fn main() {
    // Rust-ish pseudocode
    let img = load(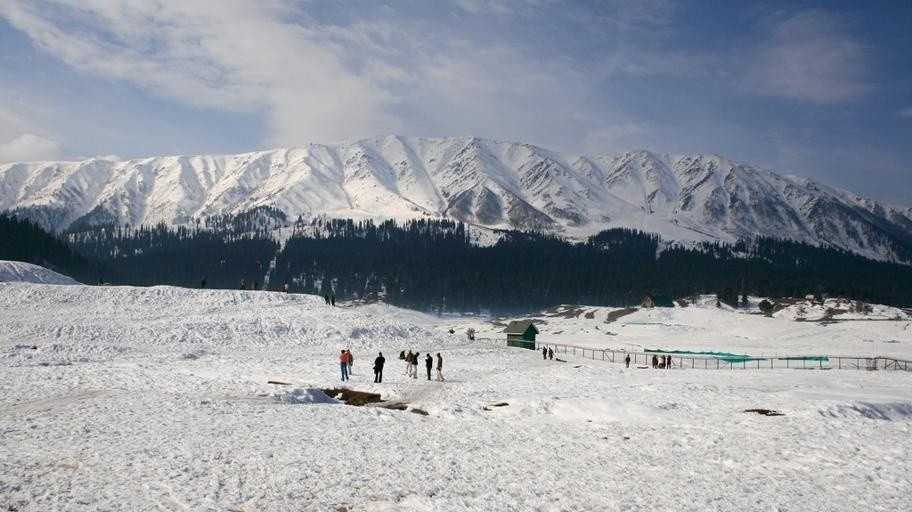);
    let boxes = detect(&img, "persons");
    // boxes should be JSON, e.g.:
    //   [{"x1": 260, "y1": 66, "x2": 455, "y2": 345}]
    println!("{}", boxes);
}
[
  {"x1": 543, "y1": 347, "x2": 547, "y2": 359},
  {"x1": 346, "y1": 349, "x2": 353, "y2": 375},
  {"x1": 374, "y1": 352, "x2": 385, "y2": 383},
  {"x1": 331, "y1": 295, "x2": 335, "y2": 305},
  {"x1": 201, "y1": 278, "x2": 205, "y2": 288},
  {"x1": 238, "y1": 278, "x2": 289, "y2": 293},
  {"x1": 435, "y1": 353, "x2": 444, "y2": 381},
  {"x1": 652, "y1": 355, "x2": 672, "y2": 369},
  {"x1": 625, "y1": 354, "x2": 629, "y2": 368},
  {"x1": 425, "y1": 355, "x2": 432, "y2": 380},
  {"x1": 339, "y1": 350, "x2": 349, "y2": 382},
  {"x1": 324, "y1": 294, "x2": 330, "y2": 305},
  {"x1": 467, "y1": 327, "x2": 475, "y2": 341},
  {"x1": 405, "y1": 350, "x2": 413, "y2": 374},
  {"x1": 409, "y1": 352, "x2": 419, "y2": 379},
  {"x1": 549, "y1": 348, "x2": 553, "y2": 360}
]
